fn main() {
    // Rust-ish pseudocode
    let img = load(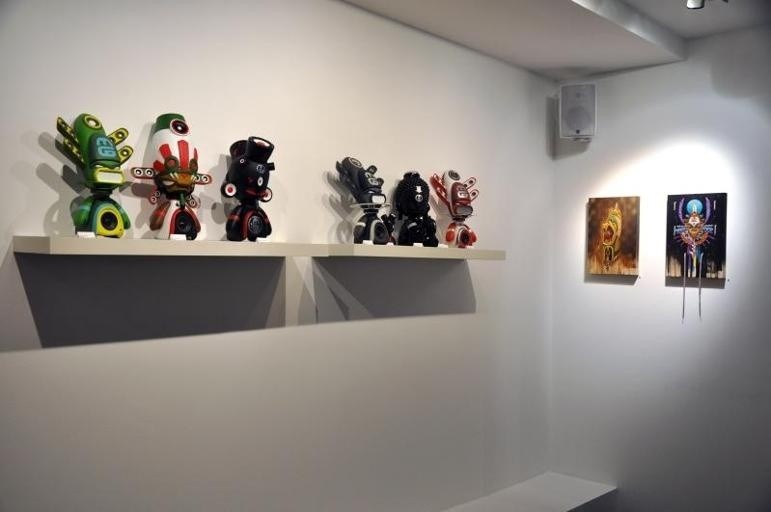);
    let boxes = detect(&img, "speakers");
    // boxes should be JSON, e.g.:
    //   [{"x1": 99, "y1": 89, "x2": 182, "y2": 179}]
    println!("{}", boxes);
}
[{"x1": 559, "y1": 83, "x2": 598, "y2": 140}]
